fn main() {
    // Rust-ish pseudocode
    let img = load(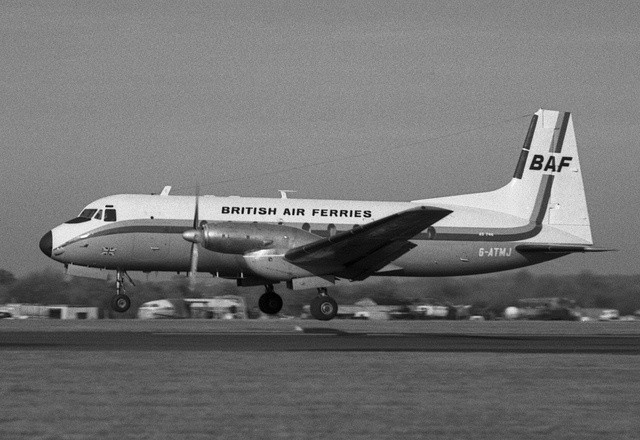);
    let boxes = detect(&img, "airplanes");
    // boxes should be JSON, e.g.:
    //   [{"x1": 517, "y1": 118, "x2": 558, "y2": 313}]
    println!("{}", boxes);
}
[{"x1": 39, "y1": 108, "x2": 617, "y2": 319}]
